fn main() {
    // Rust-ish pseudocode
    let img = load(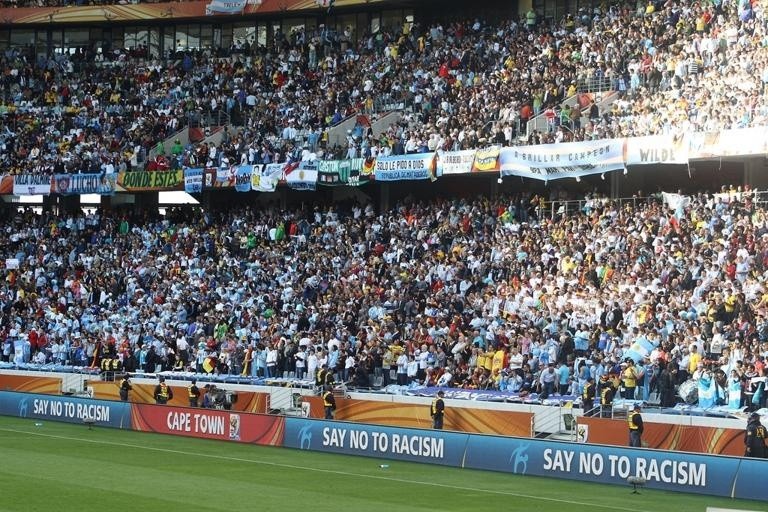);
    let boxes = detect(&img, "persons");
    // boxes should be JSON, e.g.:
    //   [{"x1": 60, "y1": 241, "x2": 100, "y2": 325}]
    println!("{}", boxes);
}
[
  {"x1": 428, "y1": 389, "x2": 446, "y2": 429},
  {"x1": 625, "y1": 404, "x2": 646, "y2": 447},
  {"x1": 1, "y1": 0, "x2": 767, "y2": 413},
  {"x1": 745, "y1": 413, "x2": 768, "y2": 458},
  {"x1": 322, "y1": 386, "x2": 336, "y2": 420}
]
